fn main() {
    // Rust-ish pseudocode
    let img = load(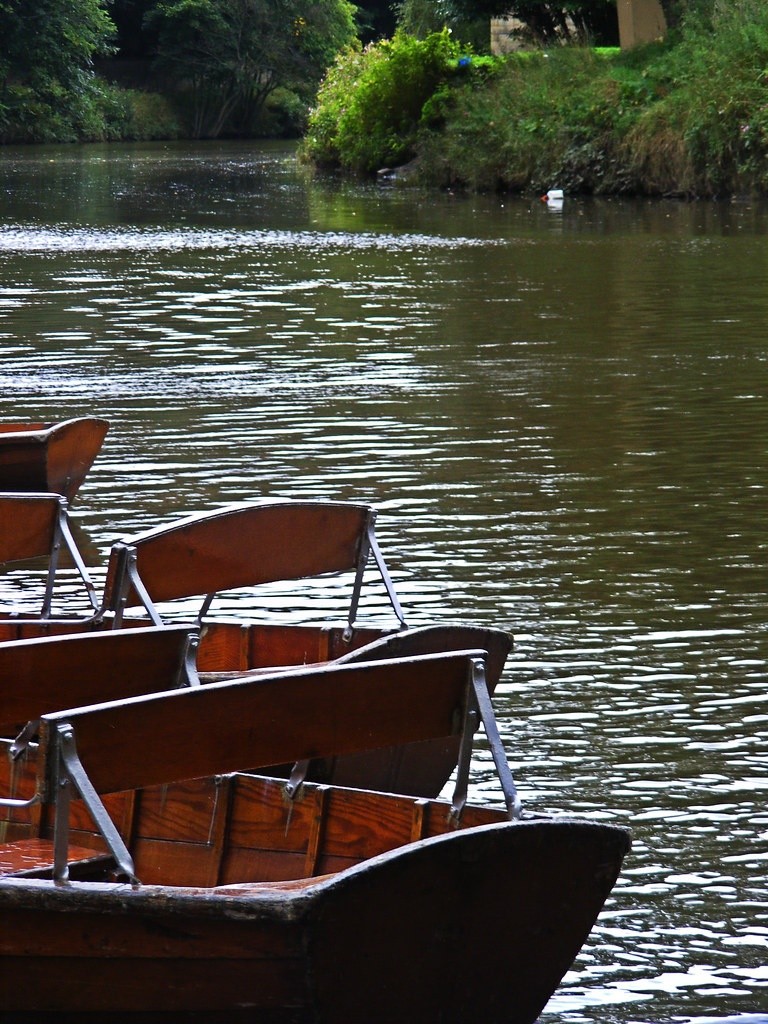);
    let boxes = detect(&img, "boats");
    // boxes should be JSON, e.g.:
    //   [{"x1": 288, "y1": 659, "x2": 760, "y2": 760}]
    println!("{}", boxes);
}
[{"x1": 1, "y1": 416, "x2": 632, "y2": 1024}]
